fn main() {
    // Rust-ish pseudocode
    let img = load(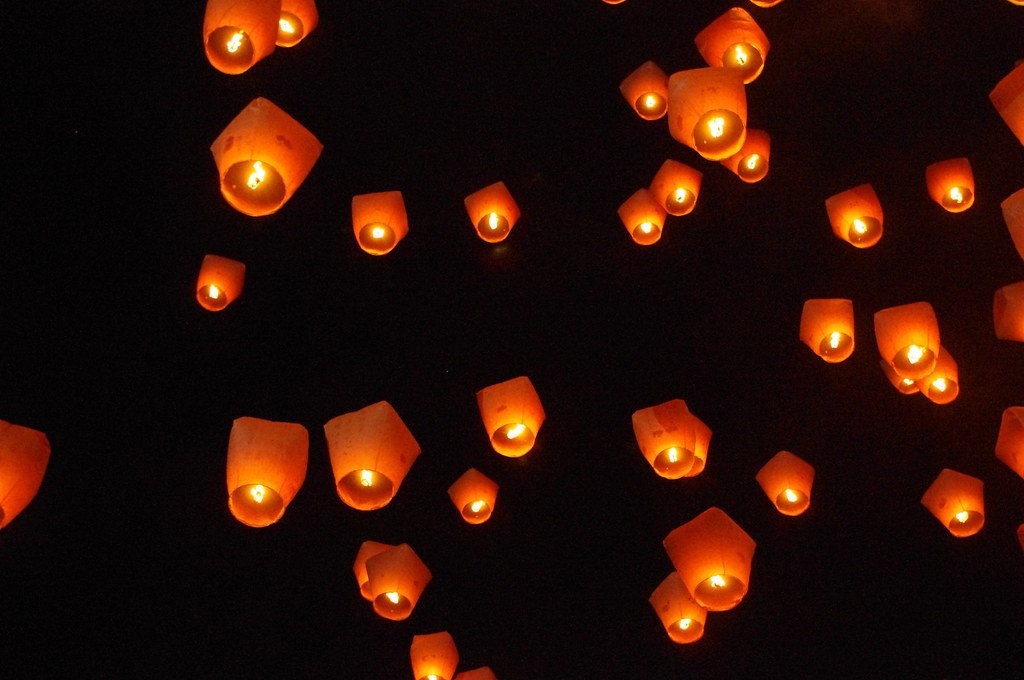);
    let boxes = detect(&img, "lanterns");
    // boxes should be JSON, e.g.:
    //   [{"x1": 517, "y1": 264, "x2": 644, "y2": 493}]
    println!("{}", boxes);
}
[{"x1": 0, "y1": 0, "x2": 1024, "y2": 680}]
